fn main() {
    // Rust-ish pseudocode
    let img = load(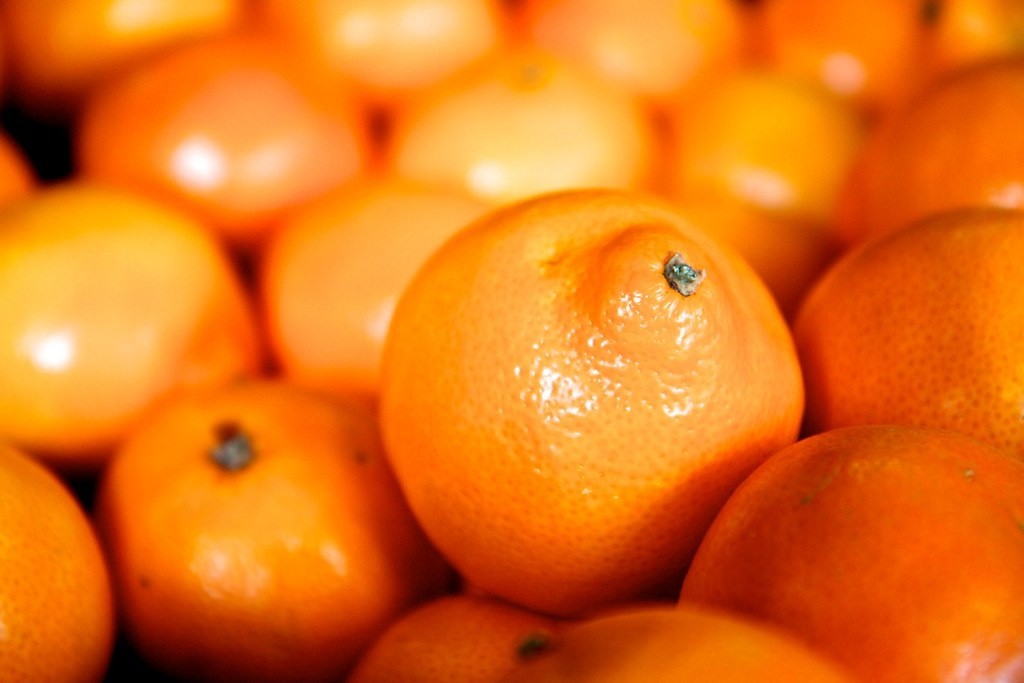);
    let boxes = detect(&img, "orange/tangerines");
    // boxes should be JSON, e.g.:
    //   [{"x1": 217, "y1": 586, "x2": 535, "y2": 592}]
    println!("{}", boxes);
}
[{"x1": 0, "y1": 0, "x2": 1024, "y2": 683}]
